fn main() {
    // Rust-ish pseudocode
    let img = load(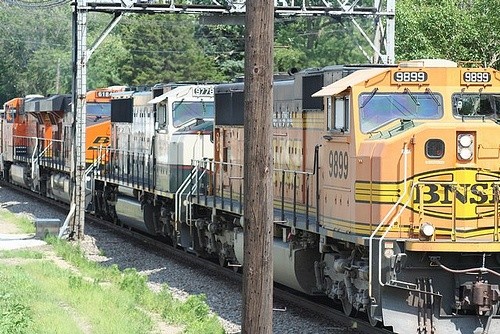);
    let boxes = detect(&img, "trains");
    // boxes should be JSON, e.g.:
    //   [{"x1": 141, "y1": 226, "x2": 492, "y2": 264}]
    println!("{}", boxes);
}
[{"x1": 1, "y1": 57, "x2": 500, "y2": 334}]
